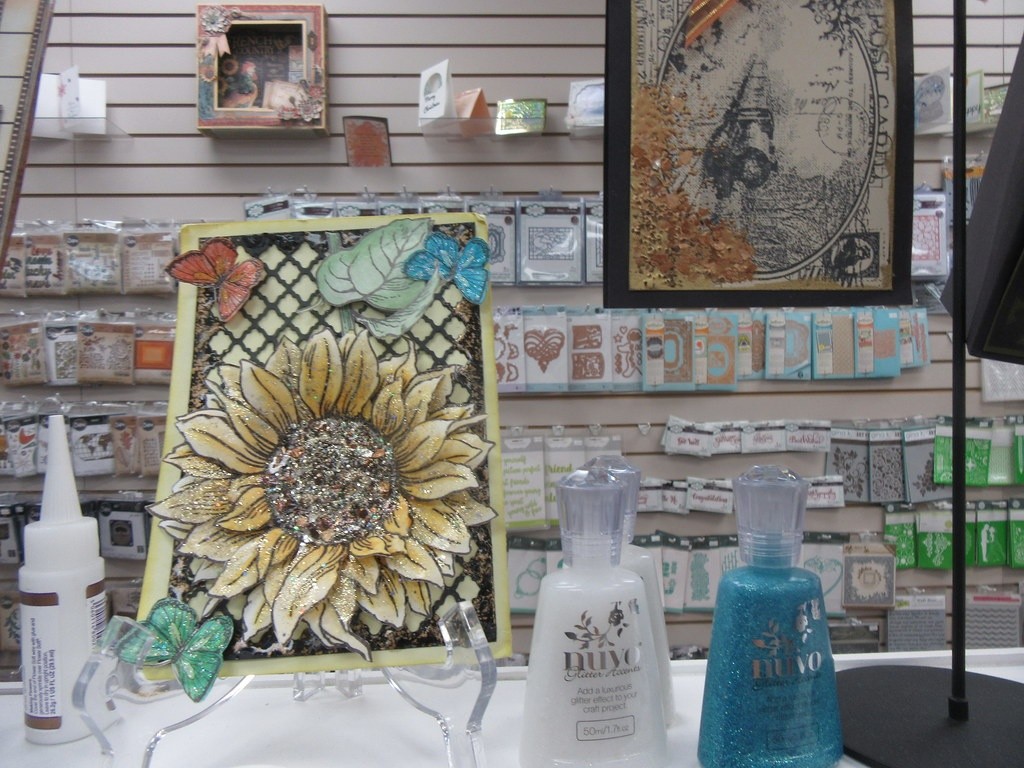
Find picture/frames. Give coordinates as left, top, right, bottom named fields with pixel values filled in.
left=603, top=0, right=916, bottom=307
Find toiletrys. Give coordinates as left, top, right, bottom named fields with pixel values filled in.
left=696, top=462, right=845, bottom=767
left=522, top=468, right=668, bottom=767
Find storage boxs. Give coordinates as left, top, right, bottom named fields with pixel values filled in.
left=196, top=3, right=331, bottom=140
